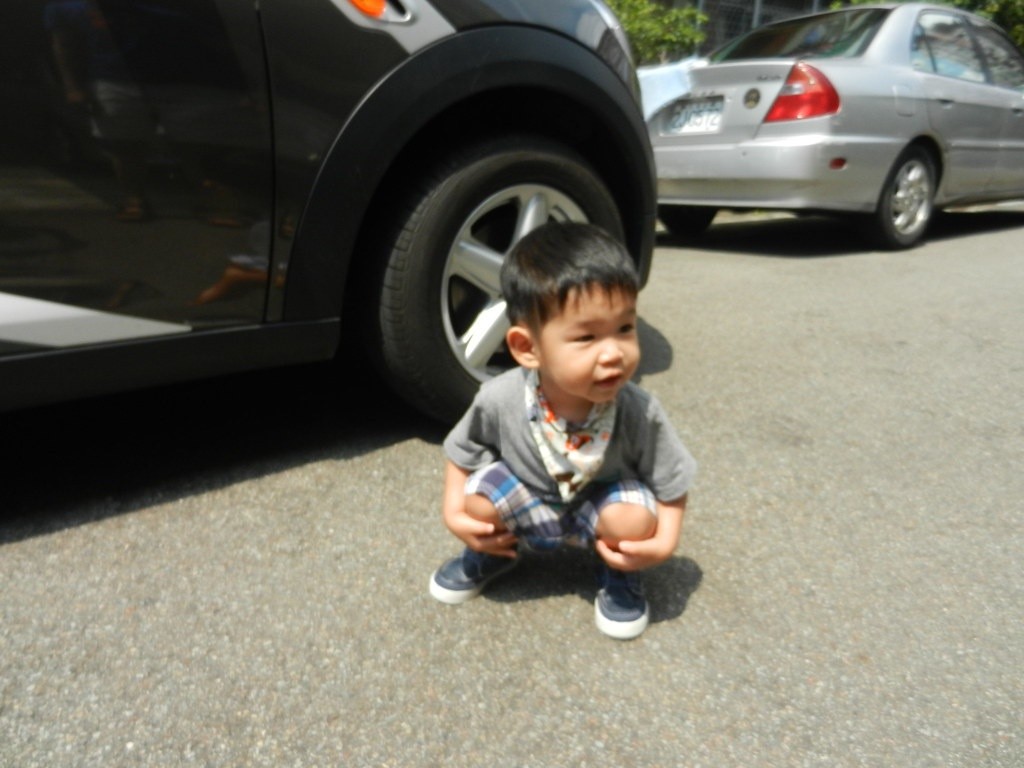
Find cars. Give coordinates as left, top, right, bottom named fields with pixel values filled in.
left=0, top=0, right=659, bottom=429
left=644, top=5, right=1024, bottom=250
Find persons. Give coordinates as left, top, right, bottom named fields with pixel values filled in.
left=429, top=221, right=689, bottom=640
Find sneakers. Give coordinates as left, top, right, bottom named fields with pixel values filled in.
left=595, top=568, right=651, bottom=639
left=429, top=543, right=521, bottom=604
